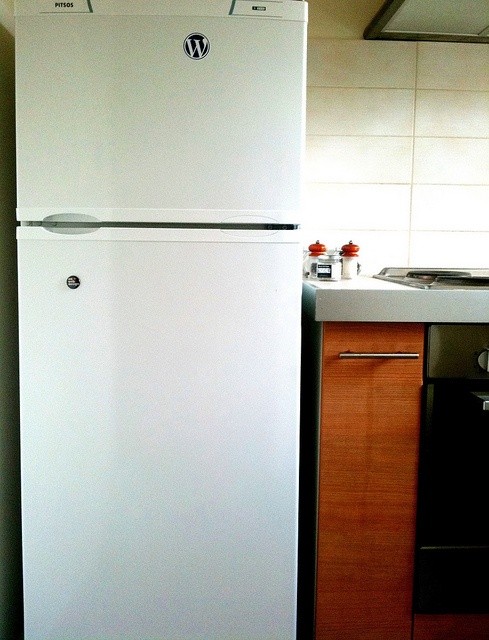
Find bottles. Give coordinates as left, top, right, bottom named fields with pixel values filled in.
left=316, top=255, right=341, bottom=282
left=308, top=240, right=327, bottom=280
left=341, top=240, right=359, bottom=280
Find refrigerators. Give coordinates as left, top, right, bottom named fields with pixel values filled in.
left=11, top=1, right=309, bottom=640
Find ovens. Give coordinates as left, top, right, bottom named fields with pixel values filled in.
left=413, top=324, right=487, bottom=616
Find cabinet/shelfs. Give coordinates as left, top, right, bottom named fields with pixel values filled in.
left=302, top=322, right=424, bottom=640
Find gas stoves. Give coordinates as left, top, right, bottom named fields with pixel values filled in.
left=406, top=270, right=489, bottom=286
